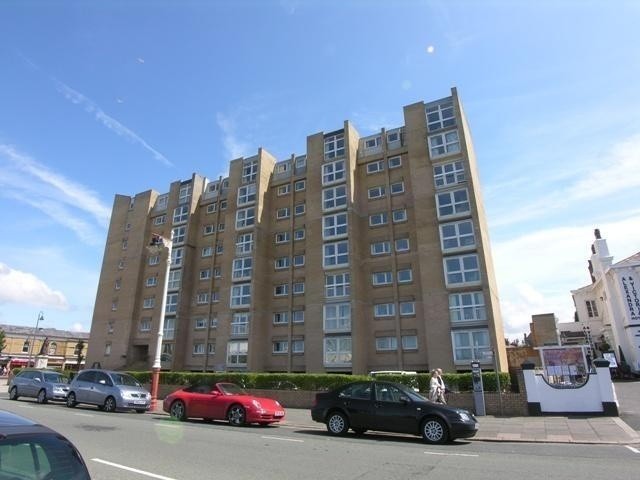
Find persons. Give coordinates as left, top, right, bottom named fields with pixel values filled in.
left=437, top=368, right=446, bottom=403
left=40, top=337, right=49, bottom=355
left=429, top=370, right=441, bottom=401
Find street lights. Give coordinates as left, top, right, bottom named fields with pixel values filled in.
left=145, top=229, right=175, bottom=411
left=27, top=311, right=45, bottom=368
left=483, top=346, right=505, bottom=418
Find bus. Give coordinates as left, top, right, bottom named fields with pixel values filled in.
left=4, top=357, right=35, bottom=375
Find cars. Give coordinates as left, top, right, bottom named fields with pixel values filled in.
left=0, top=407, right=90, bottom=479
left=66, top=368, right=152, bottom=414
left=310, top=381, right=479, bottom=444
left=8, top=367, right=70, bottom=403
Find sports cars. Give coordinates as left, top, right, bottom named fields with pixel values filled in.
left=163, top=382, right=286, bottom=427
